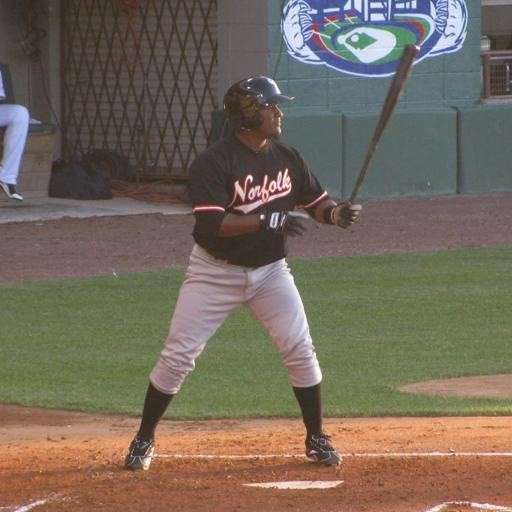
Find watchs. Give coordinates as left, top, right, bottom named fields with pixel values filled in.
left=258, top=212, right=266, bottom=232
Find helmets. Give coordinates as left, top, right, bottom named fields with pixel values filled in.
left=224, top=76, right=294, bottom=121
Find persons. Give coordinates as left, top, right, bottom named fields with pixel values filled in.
left=123, top=76, right=361, bottom=473
left=0, top=62, right=29, bottom=202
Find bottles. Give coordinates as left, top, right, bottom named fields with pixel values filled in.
left=480, top=35, right=490, bottom=51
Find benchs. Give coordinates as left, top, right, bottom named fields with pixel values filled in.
left=0, top=123, right=57, bottom=199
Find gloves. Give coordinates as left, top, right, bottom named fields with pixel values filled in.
left=333, top=200, right=361, bottom=228
left=260, top=210, right=309, bottom=237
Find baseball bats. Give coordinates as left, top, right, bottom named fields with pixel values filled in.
left=349, top=44, right=421, bottom=205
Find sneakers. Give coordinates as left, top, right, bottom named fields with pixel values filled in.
left=305, top=433, right=342, bottom=464
left=0, top=182, right=23, bottom=201
left=125, top=434, right=155, bottom=471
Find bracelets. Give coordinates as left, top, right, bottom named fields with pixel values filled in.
left=323, top=205, right=337, bottom=225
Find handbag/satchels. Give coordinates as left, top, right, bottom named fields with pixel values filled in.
left=48, top=156, right=113, bottom=200
